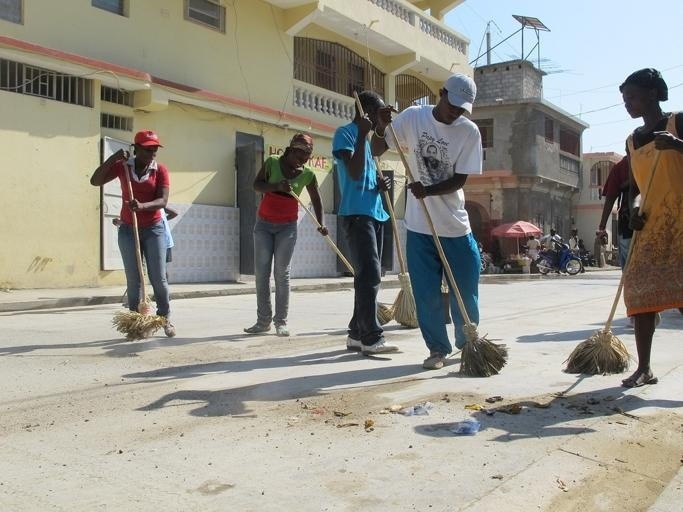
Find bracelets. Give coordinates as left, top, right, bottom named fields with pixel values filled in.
left=372, top=126, right=387, bottom=140
left=598, top=223, right=606, bottom=228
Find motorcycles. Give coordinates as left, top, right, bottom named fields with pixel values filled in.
left=475, top=241, right=489, bottom=274
left=535, top=238, right=583, bottom=276
left=579, top=249, right=597, bottom=267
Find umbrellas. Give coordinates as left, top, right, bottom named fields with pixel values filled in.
left=490, top=219, right=546, bottom=258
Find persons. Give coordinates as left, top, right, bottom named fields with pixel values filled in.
left=421, top=144, right=440, bottom=170
left=111, top=203, right=179, bottom=309
left=595, top=134, right=664, bottom=329
left=88, top=130, right=181, bottom=341
left=367, top=72, right=483, bottom=371
left=521, top=225, right=595, bottom=273
left=329, top=86, right=401, bottom=357
left=618, top=66, right=683, bottom=388
left=241, top=131, right=329, bottom=338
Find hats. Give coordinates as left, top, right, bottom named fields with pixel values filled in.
left=291, top=132, right=314, bottom=153
left=135, top=131, right=160, bottom=147
left=444, top=73, right=477, bottom=114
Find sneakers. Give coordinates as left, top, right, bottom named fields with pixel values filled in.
left=622, top=369, right=654, bottom=388
left=244, top=323, right=290, bottom=337
left=424, top=355, right=443, bottom=369
left=163, top=320, right=176, bottom=336
left=346, top=337, right=399, bottom=354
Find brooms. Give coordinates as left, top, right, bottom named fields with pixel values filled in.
left=352, top=89, right=421, bottom=330
left=387, top=122, right=510, bottom=378
left=559, top=149, right=664, bottom=376
left=289, top=189, right=395, bottom=327
left=110, top=160, right=176, bottom=343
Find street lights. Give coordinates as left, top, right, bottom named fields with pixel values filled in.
left=484, top=17, right=503, bottom=66
